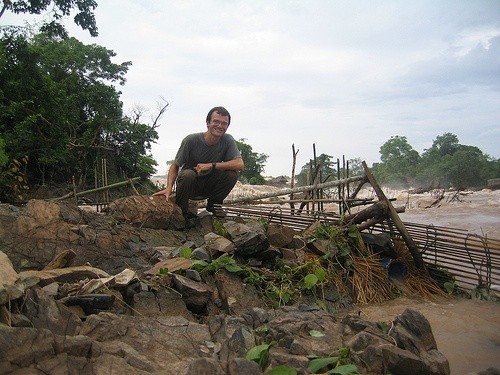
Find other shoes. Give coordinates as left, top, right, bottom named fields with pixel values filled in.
left=184, top=212, right=201, bottom=220
left=206, top=202, right=226, bottom=218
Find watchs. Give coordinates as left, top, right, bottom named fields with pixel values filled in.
left=212, top=161, right=217, bottom=171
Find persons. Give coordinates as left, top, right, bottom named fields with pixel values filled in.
left=150, top=105, right=245, bottom=227
